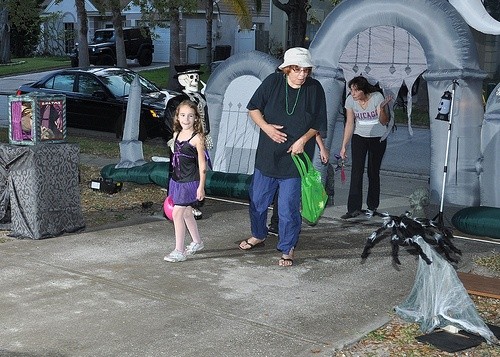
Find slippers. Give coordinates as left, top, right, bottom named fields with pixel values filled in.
left=239, top=236, right=266, bottom=250
left=280, top=257, right=293, bottom=266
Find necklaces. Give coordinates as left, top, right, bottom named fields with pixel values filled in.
left=286, top=75, right=300, bottom=115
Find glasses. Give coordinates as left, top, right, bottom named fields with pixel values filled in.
left=291, top=66, right=312, bottom=73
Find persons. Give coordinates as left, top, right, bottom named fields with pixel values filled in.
left=163, top=100, right=207, bottom=263
left=266, top=132, right=329, bottom=236
left=238, top=47, right=327, bottom=266
left=165, top=63, right=211, bottom=220
left=340, top=76, right=391, bottom=219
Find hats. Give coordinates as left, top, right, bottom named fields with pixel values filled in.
left=175, top=63, right=201, bottom=75
left=278, top=47, right=314, bottom=71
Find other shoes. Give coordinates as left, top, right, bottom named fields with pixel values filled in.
left=268, top=227, right=279, bottom=237
left=365, top=207, right=377, bottom=218
left=341, top=209, right=361, bottom=219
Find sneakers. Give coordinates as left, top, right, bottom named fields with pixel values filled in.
left=164, top=249, right=187, bottom=262
left=185, top=242, right=204, bottom=254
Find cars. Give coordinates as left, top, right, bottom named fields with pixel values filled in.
left=16, top=66, right=181, bottom=143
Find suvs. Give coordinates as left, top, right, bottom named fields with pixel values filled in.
left=71, top=26, right=155, bottom=66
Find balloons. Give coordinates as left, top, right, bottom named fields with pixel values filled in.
left=164, top=197, right=174, bottom=220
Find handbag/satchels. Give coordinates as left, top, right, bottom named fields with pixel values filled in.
left=291, top=151, right=328, bottom=226
